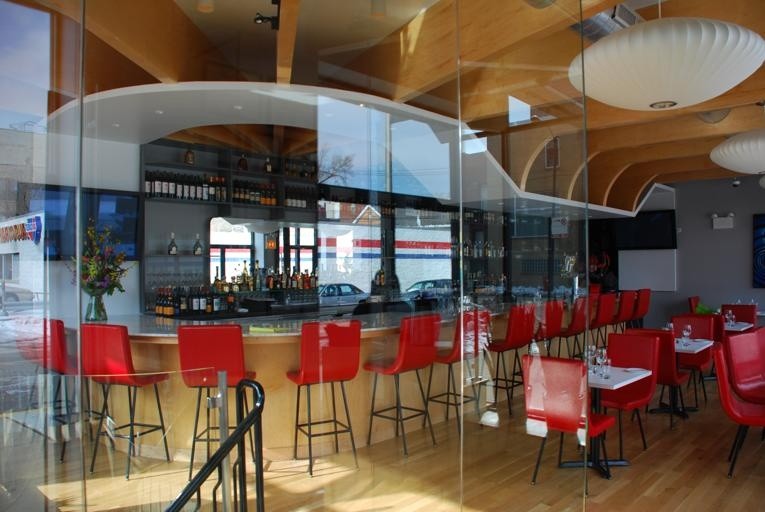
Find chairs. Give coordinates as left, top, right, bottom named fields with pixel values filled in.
left=285, top=319, right=362, bottom=477
left=360, top=311, right=441, bottom=454
left=177, top=323, right=256, bottom=481
left=12, top=315, right=94, bottom=465
left=79, top=320, right=171, bottom=482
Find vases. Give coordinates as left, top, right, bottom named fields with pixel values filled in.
left=80, top=287, right=107, bottom=323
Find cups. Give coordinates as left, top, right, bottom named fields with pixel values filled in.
left=600, top=358, right=612, bottom=376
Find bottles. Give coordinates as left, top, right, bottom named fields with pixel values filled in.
left=154, top=285, right=212, bottom=317
left=285, top=159, right=317, bottom=181
left=185, top=145, right=195, bottom=165
left=242, top=260, right=319, bottom=291
left=264, top=156, right=271, bottom=173
left=237, top=152, right=248, bottom=171
left=145, top=169, right=228, bottom=202
left=233, top=178, right=277, bottom=205
left=193, top=233, right=203, bottom=256
left=453, top=270, right=508, bottom=293
left=285, top=184, right=316, bottom=209
left=213, top=264, right=242, bottom=314
left=168, top=232, right=177, bottom=255
left=450, top=237, right=504, bottom=257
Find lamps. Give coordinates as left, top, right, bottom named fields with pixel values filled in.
left=567, top=0, right=764, bottom=111
left=710, top=127, right=765, bottom=175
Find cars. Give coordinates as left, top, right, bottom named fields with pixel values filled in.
left=317, top=283, right=371, bottom=316
left=407, top=280, right=453, bottom=300
left=0, top=286, right=33, bottom=312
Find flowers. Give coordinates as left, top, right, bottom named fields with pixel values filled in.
left=55, top=212, right=137, bottom=321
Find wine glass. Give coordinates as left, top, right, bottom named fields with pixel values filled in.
left=725, top=310, right=733, bottom=328
left=594, top=346, right=609, bottom=379
left=584, top=344, right=596, bottom=373
left=682, top=324, right=692, bottom=346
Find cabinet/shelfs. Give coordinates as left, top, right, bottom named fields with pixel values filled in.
left=456, top=209, right=509, bottom=294
left=321, top=197, right=454, bottom=300
left=509, top=213, right=553, bottom=295
left=138, top=140, right=319, bottom=313
